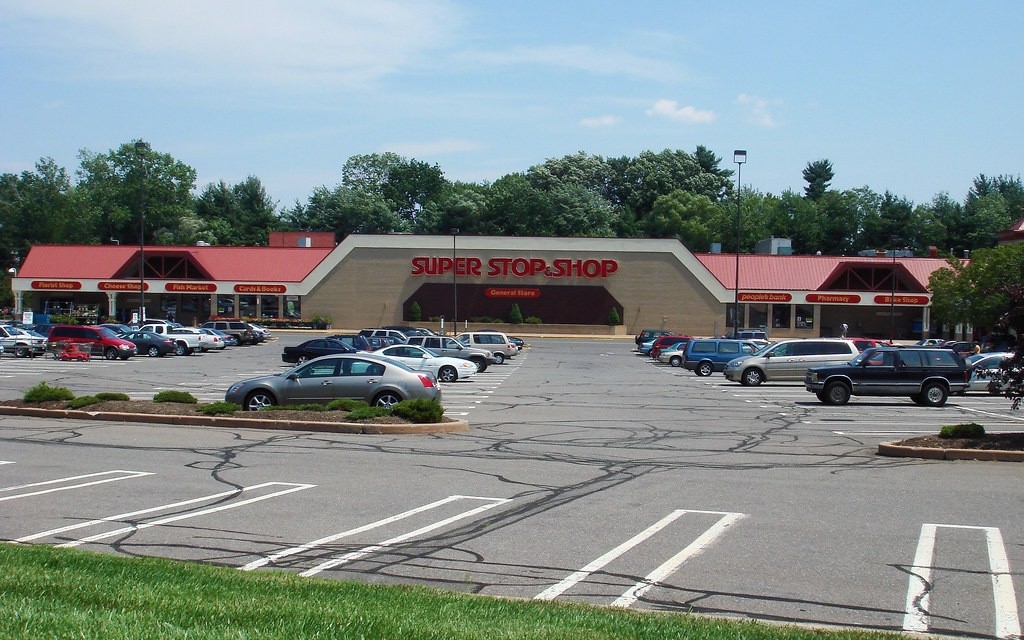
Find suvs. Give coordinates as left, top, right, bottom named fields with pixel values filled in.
left=803, top=345, right=973, bottom=405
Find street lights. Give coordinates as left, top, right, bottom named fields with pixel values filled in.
left=734, top=149, right=747, bottom=344
left=135, top=141, right=146, bottom=336
left=450, top=227, right=460, bottom=337
left=889, top=234, right=900, bottom=344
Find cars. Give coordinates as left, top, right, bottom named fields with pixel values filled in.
left=635, top=328, right=894, bottom=387
left=224, top=352, right=442, bottom=412
left=281, top=325, right=525, bottom=383
left=915, top=338, right=1024, bottom=392
left=0, top=317, right=272, bottom=358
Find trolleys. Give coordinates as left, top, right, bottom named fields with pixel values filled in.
left=45, top=338, right=95, bottom=362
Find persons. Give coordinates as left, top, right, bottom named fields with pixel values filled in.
left=840, top=321, right=848, bottom=338
left=167, top=312, right=174, bottom=323
left=970, top=341, right=980, bottom=355
